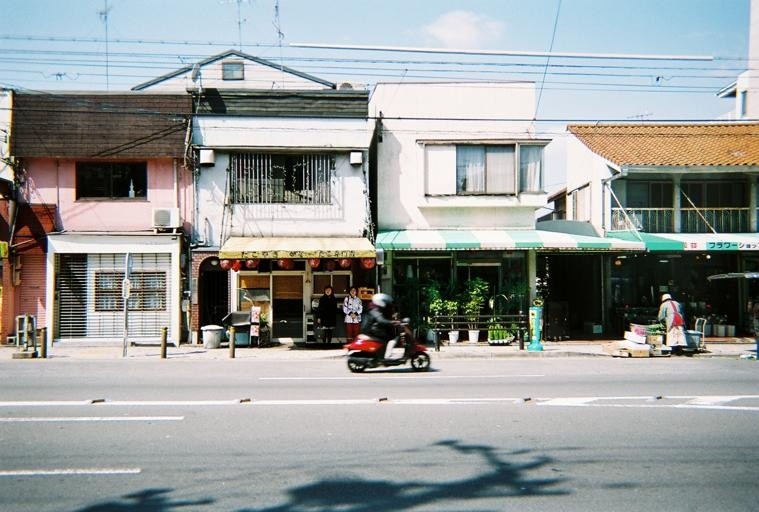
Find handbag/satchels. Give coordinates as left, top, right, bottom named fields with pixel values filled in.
left=315, top=327, right=325, bottom=338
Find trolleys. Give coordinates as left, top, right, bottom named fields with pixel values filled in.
left=680, top=317, right=708, bottom=355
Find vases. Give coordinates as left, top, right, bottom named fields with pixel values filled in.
left=697, top=322, right=736, bottom=337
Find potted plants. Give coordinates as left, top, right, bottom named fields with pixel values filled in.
left=260, top=313, right=268, bottom=328
left=392, top=271, right=531, bottom=344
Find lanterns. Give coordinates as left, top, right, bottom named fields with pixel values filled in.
left=220, top=258, right=375, bottom=272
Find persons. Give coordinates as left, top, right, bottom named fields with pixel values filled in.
left=317, top=286, right=337, bottom=344
left=752, top=302, right=759, bottom=360
left=361, top=293, right=401, bottom=360
left=657, top=293, right=689, bottom=355
left=342, top=286, right=363, bottom=341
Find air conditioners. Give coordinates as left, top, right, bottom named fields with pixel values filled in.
left=152, top=207, right=181, bottom=228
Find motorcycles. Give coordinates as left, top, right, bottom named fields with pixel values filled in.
left=343, top=311, right=433, bottom=375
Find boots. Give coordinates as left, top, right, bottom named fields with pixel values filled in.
left=385, top=341, right=397, bottom=359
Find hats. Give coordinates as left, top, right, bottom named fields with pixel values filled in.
left=661, top=293, right=671, bottom=301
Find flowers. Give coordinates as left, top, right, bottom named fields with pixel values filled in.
left=622, top=291, right=727, bottom=324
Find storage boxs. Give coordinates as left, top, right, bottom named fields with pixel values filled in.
left=646, top=335, right=664, bottom=345
left=684, top=330, right=701, bottom=347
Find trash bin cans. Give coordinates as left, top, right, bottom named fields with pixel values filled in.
left=234, top=328, right=249, bottom=346
left=200, top=325, right=224, bottom=349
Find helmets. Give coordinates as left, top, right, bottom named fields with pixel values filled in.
left=372, top=293, right=394, bottom=308
